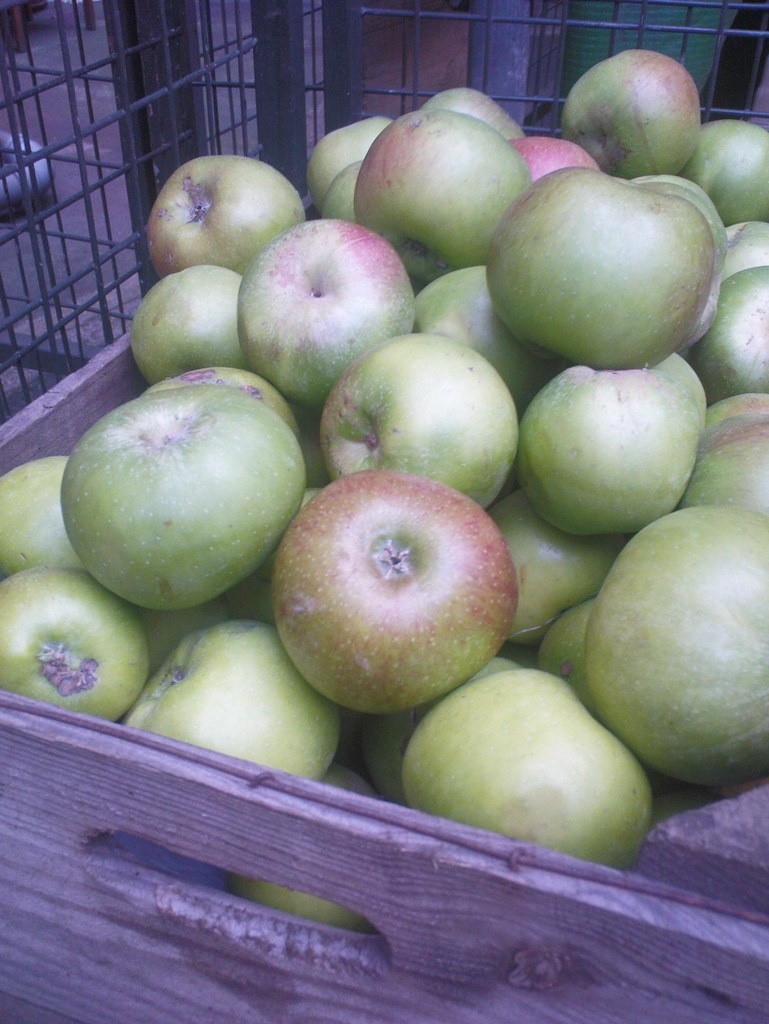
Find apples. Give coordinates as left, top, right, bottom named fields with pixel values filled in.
left=0, top=48, right=769, bottom=931
